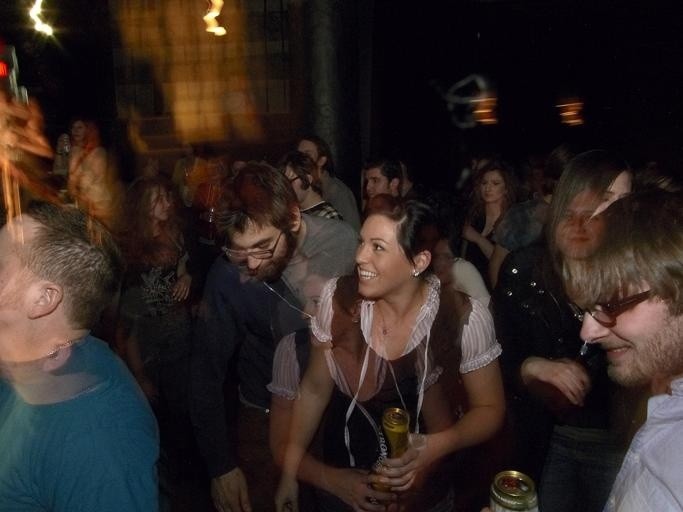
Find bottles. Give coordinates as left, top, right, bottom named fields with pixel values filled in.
left=204, top=206, right=218, bottom=241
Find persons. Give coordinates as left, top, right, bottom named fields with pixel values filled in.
left=1, top=85, right=683, bottom=512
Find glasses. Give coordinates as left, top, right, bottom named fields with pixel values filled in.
left=567, top=289, right=657, bottom=327
left=221, top=231, right=283, bottom=260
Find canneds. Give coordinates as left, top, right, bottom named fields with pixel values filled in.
left=379, top=407, right=410, bottom=458
left=488, top=471, right=543, bottom=512
